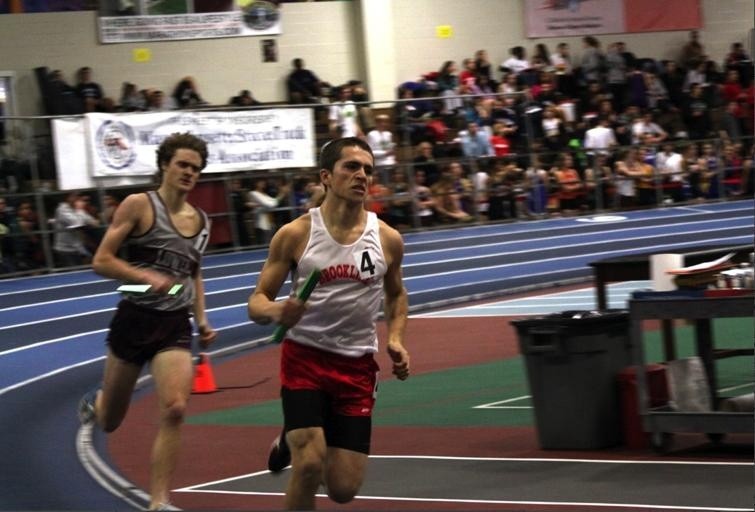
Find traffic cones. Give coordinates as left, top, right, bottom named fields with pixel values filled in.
left=190, top=350, right=220, bottom=395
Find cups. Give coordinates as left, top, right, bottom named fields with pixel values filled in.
left=648, top=251, right=683, bottom=291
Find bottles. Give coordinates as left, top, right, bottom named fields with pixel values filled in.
left=713, top=262, right=755, bottom=288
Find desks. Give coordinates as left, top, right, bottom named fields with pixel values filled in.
left=587, top=243, right=755, bottom=442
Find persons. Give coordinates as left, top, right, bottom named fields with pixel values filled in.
left=77, top=132, right=218, bottom=511
left=248, top=139, right=411, bottom=511
left=0, top=28, right=755, bottom=269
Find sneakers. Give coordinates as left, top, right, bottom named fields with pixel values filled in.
left=267, top=428, right=291, bottom=471
left=77, top=384, right=103, bottom=424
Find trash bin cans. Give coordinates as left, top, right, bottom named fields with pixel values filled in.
left=508, top=309, right=631, bottom=451
